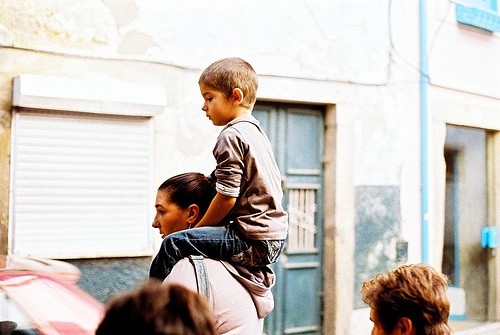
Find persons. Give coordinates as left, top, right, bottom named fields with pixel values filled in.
left=150, top=170, right=277, bottom=334
left=146, top=56, right=291, bottom=287
left=359, top=263, right=453, bottom=335
left=94, top=281, right=221, bottom=335
